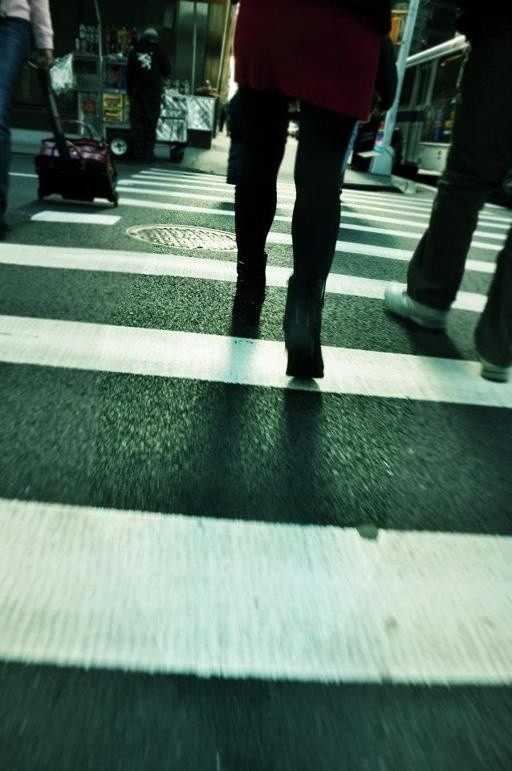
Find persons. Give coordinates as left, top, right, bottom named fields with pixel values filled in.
left=377, top=0, right=512, bottom=384
left=192, top=80, right=221, bottom=99
left=104, top=37, right=139, bottom=91
left=220, top=3, right=401, bottom=380
left=0, top=0, right=60, bottom=243
left=123, top=26, right=171, bottom=170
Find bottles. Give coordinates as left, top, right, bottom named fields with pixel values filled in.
left=77, top=23, right=143, bottom=60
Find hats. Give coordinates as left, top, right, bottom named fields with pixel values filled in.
left=144, top=27, right=159, bottom=41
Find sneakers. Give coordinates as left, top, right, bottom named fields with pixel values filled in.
left=383, top=280, right=451, bottom=330
left=480, top=356, right=511, bottom=384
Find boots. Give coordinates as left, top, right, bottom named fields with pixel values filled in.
left=280, top=270, right=327, bottom=379
left=233, top=249, right=267, bottom=307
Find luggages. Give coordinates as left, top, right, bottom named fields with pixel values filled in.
left=25, top=56, right=120, bottom=207
